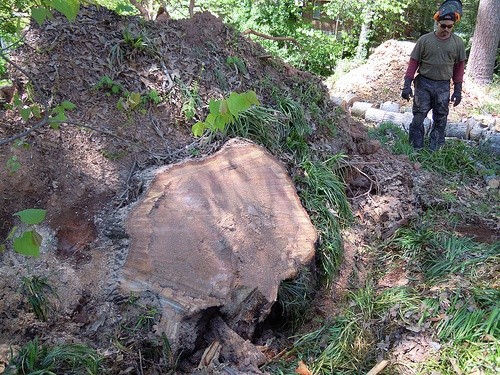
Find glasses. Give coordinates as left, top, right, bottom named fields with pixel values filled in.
left=438, top=21, right=455, bottom=29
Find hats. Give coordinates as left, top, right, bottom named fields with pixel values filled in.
left=433, top=0, right=463, bottom=22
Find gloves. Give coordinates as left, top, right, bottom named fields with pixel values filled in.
left=451, top=82, right=462, bottom=107
left=401, top=78, right=414, bottom=102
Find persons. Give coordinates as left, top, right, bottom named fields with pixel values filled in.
left=401, top=0, right=467, bottom=151
